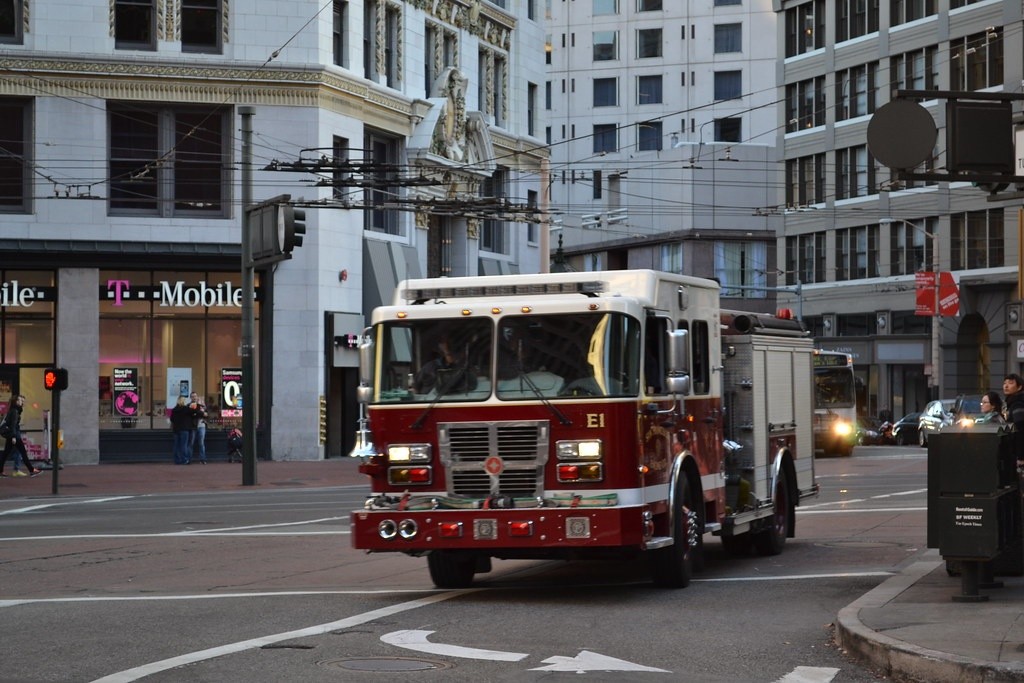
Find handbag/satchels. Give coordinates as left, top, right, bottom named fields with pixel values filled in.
left=0, top=423, right=10, bottom=438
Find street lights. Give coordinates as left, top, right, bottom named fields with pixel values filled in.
left=877, top=216, right=943, bottom=399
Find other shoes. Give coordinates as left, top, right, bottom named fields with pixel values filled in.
left=199, top=460, right=206, bottom=463
left=185, top=461, right=191, bottom=464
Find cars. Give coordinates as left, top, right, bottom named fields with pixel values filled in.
left=892, top=411, right=923, bottom=445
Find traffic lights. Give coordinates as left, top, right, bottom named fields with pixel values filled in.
left=43, top=368, right=69, bottom=391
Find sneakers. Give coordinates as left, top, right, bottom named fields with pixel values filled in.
left=30, top=470, right=43, bottom=477
left=12, top=472, right=27, bottom=477
left=1, top=473, right=9, bottom=478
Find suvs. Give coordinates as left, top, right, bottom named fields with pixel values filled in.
left=918, top=399, right=955, bottom=446
left=949, top=393, right=986, bottom=427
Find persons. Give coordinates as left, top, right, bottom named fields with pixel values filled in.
left=410, top=330, right=477, bottom=396
left=979, top=391, right=1007, bottom=425
left=0, top=395, right=44, bottom=478
left=7, top=395, right=27, bottom=477
left=878, top=403, right=894, bottom=425
left=170, top=396, right=208, bottom=465
left=185, top=391, right=208, bottom=465
left=1002, top=373, right=1024, bottom=433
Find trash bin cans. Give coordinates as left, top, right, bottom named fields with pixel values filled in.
left=926, top=422, right=1016, bottom=602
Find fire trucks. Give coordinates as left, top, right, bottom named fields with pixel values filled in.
left=347, top=266, right=821, bottom=591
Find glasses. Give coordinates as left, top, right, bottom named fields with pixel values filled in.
left=981, top=401, right=990, bottom=403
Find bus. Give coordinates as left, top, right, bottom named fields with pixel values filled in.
left=811, top=349, right=863, bottom=458
